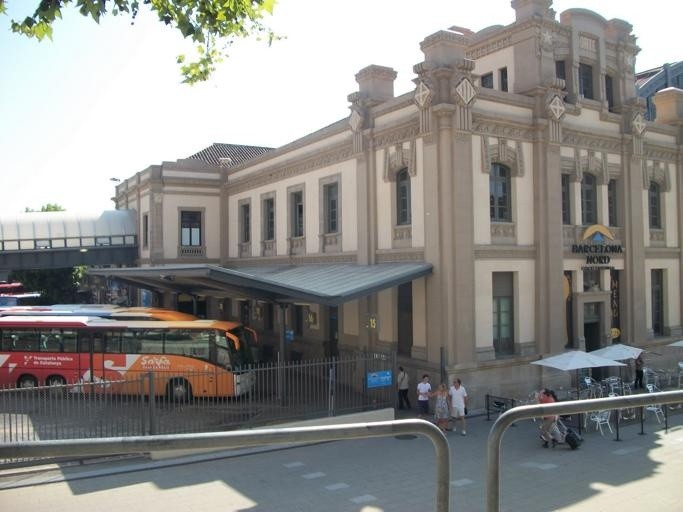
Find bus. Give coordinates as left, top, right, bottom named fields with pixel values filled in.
left=0, top=314, right=261, bottom=406
left=0, top=291, right=46, bottom=307
left=0, top=280, right=25, bottom=294
left=0, top=302, right=197, bottom=322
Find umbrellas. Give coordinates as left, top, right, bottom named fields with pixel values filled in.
left=665, top=340, right=683, bottom=347
left=528, top=350, right=628, bottom=434
left=587, top=344, right=645, bottom=394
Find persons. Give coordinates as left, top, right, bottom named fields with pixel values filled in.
left=396, top=366, right=411, bottom=410
left=415, top=375, right=432, bottom=420
left=535, top=388, right=557, bottom=448
left=449, top=378, right=468, bottom=436
left=547, top=390, right=561, bottom=444
left=635, top=355, right=644, bottom=389
left=432, top=383, right=450, bottom=433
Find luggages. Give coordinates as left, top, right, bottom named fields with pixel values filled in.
left=558, top=419, right=582, bottom=449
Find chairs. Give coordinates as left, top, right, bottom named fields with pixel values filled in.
left=504, top=360, right=682, bottom=437
